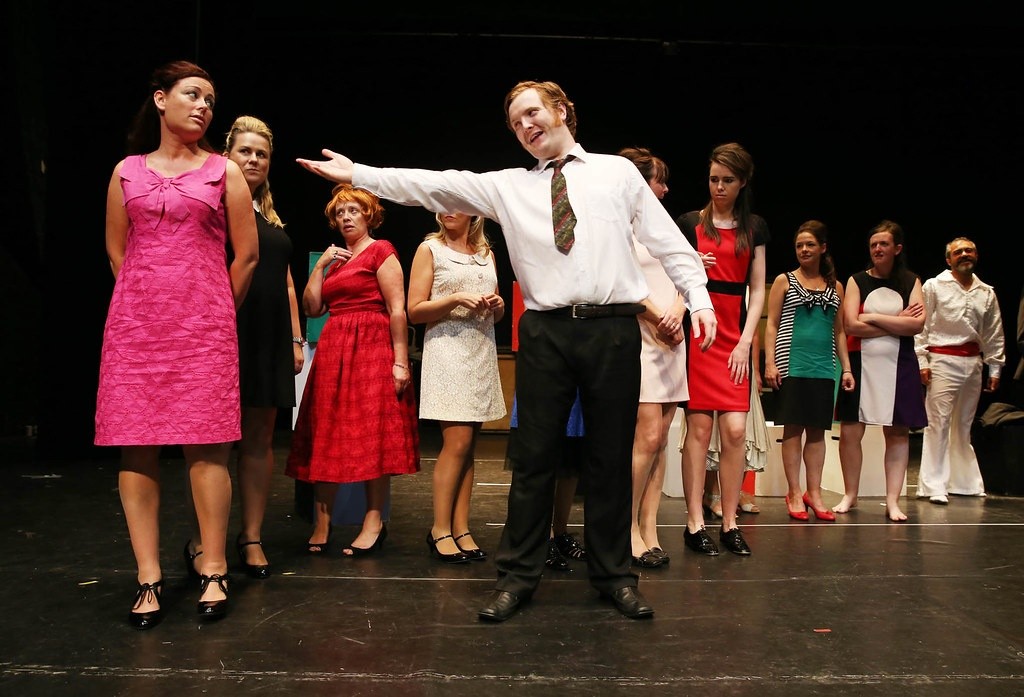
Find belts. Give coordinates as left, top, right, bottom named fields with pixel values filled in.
left=555, top=302, right=647, bottom=319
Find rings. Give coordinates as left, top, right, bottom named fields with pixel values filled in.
left=335, top=251, right=338, bottom=254
left=334, top=255, right=336, bottom=258
left=672, top=325, right=675, bottom=328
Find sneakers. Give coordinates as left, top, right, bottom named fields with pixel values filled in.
left=555, top=533, right=588, bottom=560
left=545, top=538, right=569, bottom=568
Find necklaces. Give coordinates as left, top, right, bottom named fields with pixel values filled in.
left=798, top=268, right=823, bottom=292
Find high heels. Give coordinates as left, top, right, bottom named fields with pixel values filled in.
left=802, top=491, right=836, bottom=521
left=306, top=523, right=332, bottom=553
left=197, top=570, right=230, bottom=615
left=236, top=532, right=271, bottom=578
left=453, top=532, right=486, bottom=559
left=738, top=491, right=760, bottom=513
left=702, top=492, right=739, bottom=518
left=342, top=522, right=387, bottom=558
left=786, top=495, right=810, bottom=520
left=184, top=540, right=206, bottom=578
left=426, top=532, right=471, bottom=562
left=128, top=574, right=164, bottom=629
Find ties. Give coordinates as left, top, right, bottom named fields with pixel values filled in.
left=546, top=155, right=577, bottom=255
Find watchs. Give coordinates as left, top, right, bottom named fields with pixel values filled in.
left=292, top=337, right=304, bottom=344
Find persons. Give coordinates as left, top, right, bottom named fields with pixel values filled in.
left=283, top=182, right=422, bottom=554
left=186, top=114, right=303, bottom=579
left=296, top=80, right=718, bottom=623
left=93, top=62, right=260, bottom=631
left=406, top=205, right=508, bottom=564
left=678, top=285, right=773, bottom=520
left=509, top=379, right=585, bottom=571
left=831, top=219, right=927, bottom=521
left=613, top=146, right=692, bottom=568
left=915, top=234, right=1006, bottom=504
left=676, top=143, right=766, bottom=553
left=765, top=219, right=855, bottom=522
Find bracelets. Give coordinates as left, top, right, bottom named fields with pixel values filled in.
left=843, top=371, right=852, bottom=373
left=394, top=363, right=410, bottom=370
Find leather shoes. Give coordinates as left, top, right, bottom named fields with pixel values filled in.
left=599, top=586, right=655, bottom=617
left=649, top=547, right=670, bottom=561
left=477, top=590, right=523, bottom=620
left=632, top=551, right=662, bottom=567
left=684, top=526, right=720, bottom=555
left=720, top=527, right=751, bottom=554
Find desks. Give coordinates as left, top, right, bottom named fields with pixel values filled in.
left=482, top=353, right=518, bottom=435
